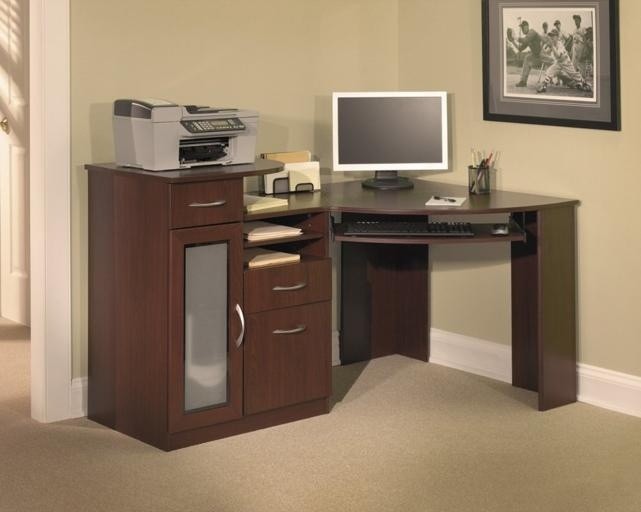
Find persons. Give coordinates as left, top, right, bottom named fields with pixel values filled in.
left=505, top=11, right=594, bottom=98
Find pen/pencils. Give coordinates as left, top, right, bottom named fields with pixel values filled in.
left=470, top=151, right=493, bottom=195
left=434, top=196, right=456, bottom=202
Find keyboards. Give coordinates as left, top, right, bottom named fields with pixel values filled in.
left=346, top=221, right=475, bottom=236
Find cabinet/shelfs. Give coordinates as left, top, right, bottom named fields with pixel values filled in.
left=82, top=156, right=331, bottom=452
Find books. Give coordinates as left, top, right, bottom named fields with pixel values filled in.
left=240, top=219, right=304, bottom=242
left=243, top=247, right=303, bottom=270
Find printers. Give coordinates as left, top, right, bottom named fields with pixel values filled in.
left=111, top=96, right=261, bottom=172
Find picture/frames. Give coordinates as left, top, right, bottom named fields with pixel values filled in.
left=480, top=0, right=619, bottom=133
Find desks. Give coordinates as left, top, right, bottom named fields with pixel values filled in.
left=333, top=175, right=580, bottom=413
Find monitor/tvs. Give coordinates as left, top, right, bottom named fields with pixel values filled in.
left=332, top=90, right=450, bottom=190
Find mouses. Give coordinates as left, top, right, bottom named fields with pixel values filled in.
left=489, top=221, right=509, bottom=236
